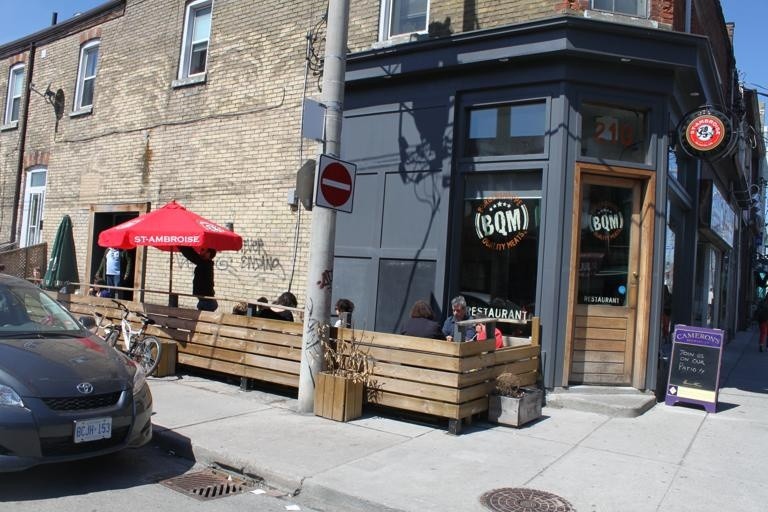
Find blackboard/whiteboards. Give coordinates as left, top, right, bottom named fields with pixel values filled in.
left=665, top=324, right=724, bottom=415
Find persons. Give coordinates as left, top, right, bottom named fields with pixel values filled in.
left=59, top=246, right=132, bottom=298
left=255, top=297, right=268, bottom=317
left=708, top=287, right=714, bottom=320
left=472, top=312, right=504, bottom=349
left=233, top=300, right=247, bottom=315
left=33, top=266, right=42, bottom=288
left=177, top=245, right=218, bottom=311
left=400, top=300, right=442, bottom=339
left=756, top=293, right=768, bottom=352
left=260, top=292, right=297, bottom=322
left=334, top=298, right=354, bottom=327
left=442, top=296, right=476, bottom=343
left=0, top=264, right=6, bottom=274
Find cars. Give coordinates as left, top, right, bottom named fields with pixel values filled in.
left=580, top=269, right=626, bottom=305
left=459, top=290, right=533, bottom=342
left=0, top=272, right=156, bottom=474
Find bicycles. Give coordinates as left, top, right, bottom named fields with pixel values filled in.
left=88, top=309, right=113, bottom=340
left=107, top=298, right=163, bottom=377
left=23, top=299, right=72, bottom=330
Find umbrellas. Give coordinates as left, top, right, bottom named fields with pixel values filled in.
left=97, top=198, right=250, bottom=329
left=40, top=215, right=80, bottom=290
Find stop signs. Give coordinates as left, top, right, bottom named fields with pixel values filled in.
left=314, top=153, right=357, bottom=214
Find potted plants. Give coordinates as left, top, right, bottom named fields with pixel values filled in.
left=304, top=319, right=374, bottom=422
left=487, top=371, right=544, bottom=428
